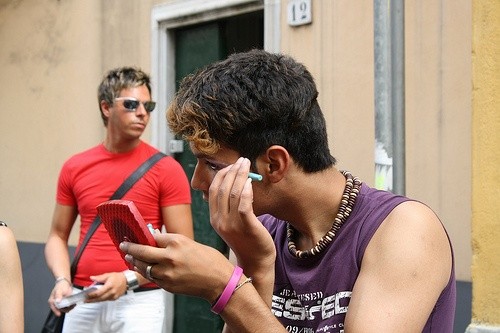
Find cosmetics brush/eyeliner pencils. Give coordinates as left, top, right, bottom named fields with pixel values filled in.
left=217, top=162, right=262, bottom=181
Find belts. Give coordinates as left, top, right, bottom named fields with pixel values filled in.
left=73, top=285, right=161, bottom=294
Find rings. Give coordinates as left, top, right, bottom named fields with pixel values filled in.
left=146, top=263, right=152, bottom=281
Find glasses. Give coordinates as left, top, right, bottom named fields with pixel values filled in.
left=115, top=97, right=156, bottom=112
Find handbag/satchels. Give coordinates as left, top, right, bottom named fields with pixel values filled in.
left=41, top=307, right=68, bottom=333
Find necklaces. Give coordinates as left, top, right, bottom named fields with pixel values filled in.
left=287, top=170, right=361, bottom=261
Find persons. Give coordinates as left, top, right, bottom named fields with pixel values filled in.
left=0, top=221, right=25, bottom=333
left=120, top=49, right=457, bottom=333
left=43, top=66, right=194, bottom=333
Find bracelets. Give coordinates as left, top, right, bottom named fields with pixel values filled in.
left=124, top=269, right=140, bottom=290
left=55, top=277, right=72, bottom=287
left=211, top=266, right=251, bottom=314
